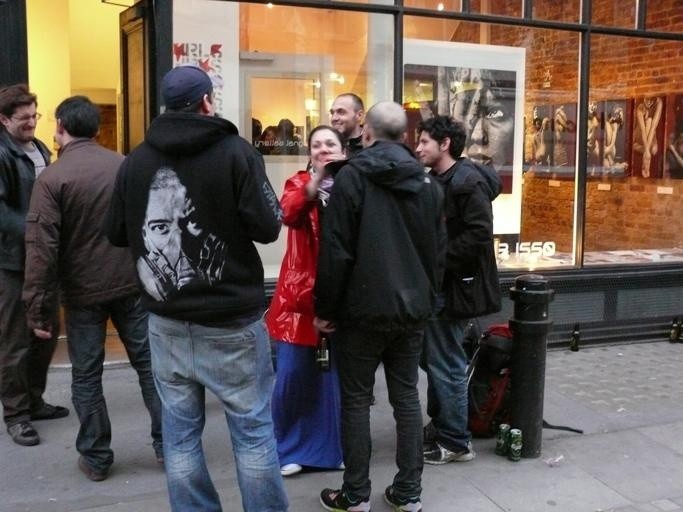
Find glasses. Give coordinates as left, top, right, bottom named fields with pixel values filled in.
left=13, top=112, right=43, bottom=122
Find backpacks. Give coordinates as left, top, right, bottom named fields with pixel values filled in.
left=465, top=323, right=514, bottom=437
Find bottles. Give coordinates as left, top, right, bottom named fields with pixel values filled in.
left=669, top=318, right=678, bottom=342
left=315, top=329, right=331, bottom=371
left=569, top=323, right=581, bottom=351
left=678, top=320, right=683, bottom=342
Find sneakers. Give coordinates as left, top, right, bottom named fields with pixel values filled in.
left=8, top=422, right=40, bottom=446
left=422, top=423, right=438, bottom=443
left=424, top=441, right=476, bottom=464
left=31, top=404, right=69, bottom=421
left=384, top=486, right=423, bottom=512
left=320, top=488, right=371, bottom=511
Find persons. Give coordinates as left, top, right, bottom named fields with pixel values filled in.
left=269, top=92, right=365, bottom=473
left=107, top=66, right=291, bottom=510
left=415, top=117, right=501, bottom=466
left=252, top=117, right=298, bottom=154
left=447, top=68, right=513, bottom=181
left=313, top=101, right=439, bottom=512
left=532, top=98, right=664, bottom=178
left=22, top=96, right=164, bottom=480
left=0, top=82, right=70, bottom=447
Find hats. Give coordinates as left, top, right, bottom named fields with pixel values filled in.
left=161, top=66, right=212, bottom=110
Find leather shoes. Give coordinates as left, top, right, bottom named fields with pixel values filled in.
left=78, top=456, right=106, bottom=480
left=280, top=463, right=302, bottom=476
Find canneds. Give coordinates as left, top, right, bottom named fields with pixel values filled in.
left=509, top=429, right=523, bottom=462
left=496, top=424, right=511, bottom=456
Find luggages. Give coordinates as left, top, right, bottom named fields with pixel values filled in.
left=422, top=319, right=482, bottom=415
